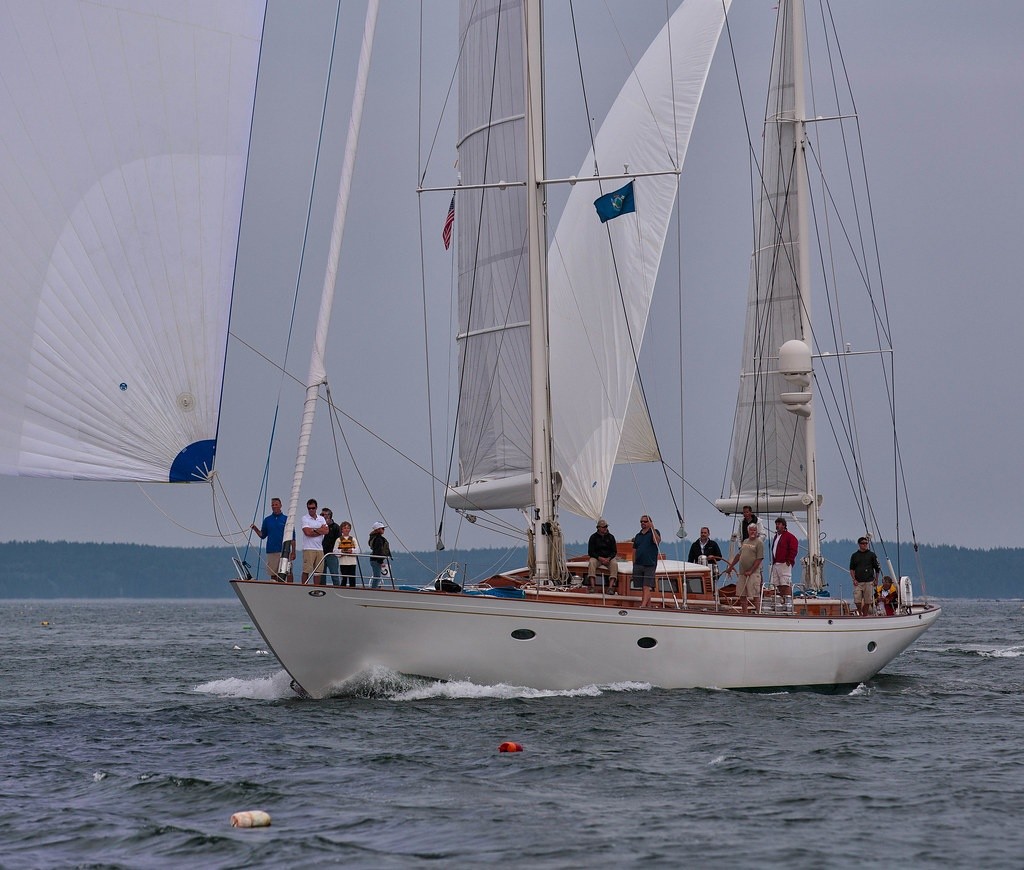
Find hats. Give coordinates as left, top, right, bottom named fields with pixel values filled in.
left=596, top=520, right=609, bottom=528
left=858, top=537, right=867, bottom=543
left=371, top=522, right=386, bottom=530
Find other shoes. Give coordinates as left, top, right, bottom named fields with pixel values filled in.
left=607, top=588, right=614, bottom=594
left=589, top=588, right=594, bottom=593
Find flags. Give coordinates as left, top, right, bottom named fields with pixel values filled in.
left=594, top=180, right=637, bottom=223
left=442, top=196, right=455, bottom=250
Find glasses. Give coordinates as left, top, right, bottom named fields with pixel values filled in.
left=320, top=513, right=329, bottom=517
left=859, top=542, right=867, bottom=544
left=380, top=528, right=384, bottom=530
left=640, top=521, right=649, bottom=523
left=602, top=526, right=607, bottom=528
left=308, top=507, right=315, bottom=510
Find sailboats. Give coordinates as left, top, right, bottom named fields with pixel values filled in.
left=0, top=3, right=943, bottom=697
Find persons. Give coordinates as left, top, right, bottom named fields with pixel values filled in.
left=870, top=576, right=898, bottom=616
left=769, top=517, right=799, bottom=604
left=368, top=522, right=389, bottom=588
left=726, top=523, right=765, bottom=614
left=688, top=527, right=722, bottom=581
left=632, top=515, right=661, bottom=608
left=850, top=537, right=880, bottom=616
left=588, top=520, right=618, bottom=595
left=737, top=505, right=766, bottom=582
left=320, top=508, right=340, bottom=586
left=301, top=499, right=329, bottom=584
left=333, top=522, right=360, bottom=587
left=251, top=497, right=297, bottom=582
